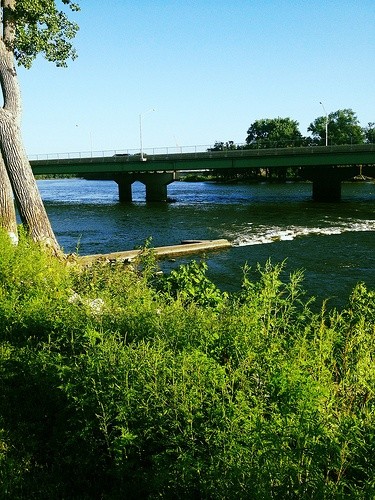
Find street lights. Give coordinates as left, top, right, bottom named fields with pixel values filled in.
left=140, top=109, right=156, bottom=160
left=319, top=101, right=328, bottom=146
